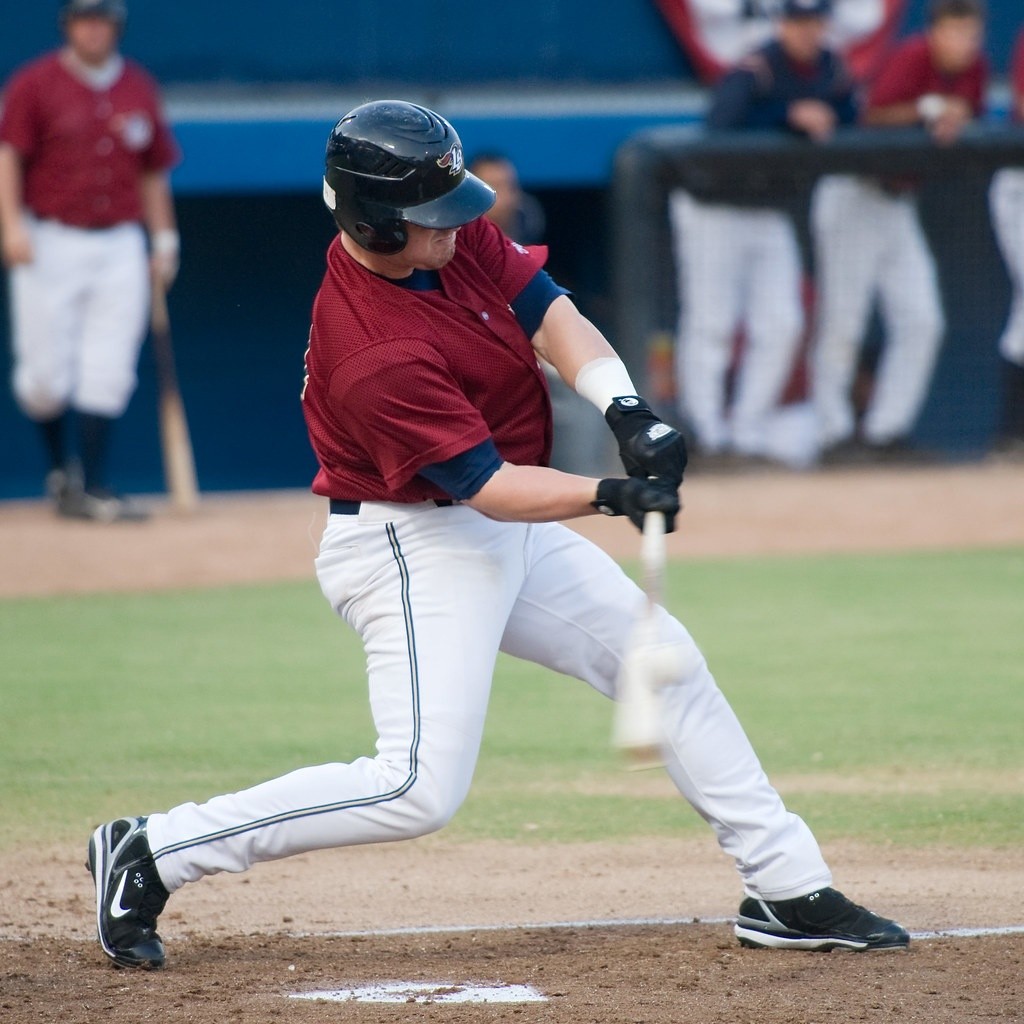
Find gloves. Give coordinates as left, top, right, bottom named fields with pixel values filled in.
left=604, top=393, right=688, bottom=489
left=591, top=475, right=681, bottom=533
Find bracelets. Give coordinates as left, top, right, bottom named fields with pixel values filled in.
left=151, top=231, right=181, bottom=253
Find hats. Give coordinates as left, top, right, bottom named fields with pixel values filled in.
left=779, top=1, right=831, bottom=19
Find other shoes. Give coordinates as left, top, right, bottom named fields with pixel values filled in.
left=46, top=454, right=138, bottom=519
left=816, top=434, right=927, bottom=466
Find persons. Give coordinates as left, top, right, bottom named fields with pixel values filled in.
left=809, top=0, right=1024, bottom=468
left=89, top=98, right=911, bottom=969
left=668, top=0, right=863, bottom=464
left=470, top=150, right=544, bottom=252
left=0, top=3, right=181, bottom=521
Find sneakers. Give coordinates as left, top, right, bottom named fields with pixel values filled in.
left=733, top=887, right=910, bottom=953
left=83, top=815, right=170, bottom=971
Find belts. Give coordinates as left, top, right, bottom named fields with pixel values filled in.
left=329, top=497, right=453, bottom=515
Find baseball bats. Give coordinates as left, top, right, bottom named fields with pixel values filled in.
left=612, top=509, right=674, bottom=766
left=147, top=266, right=201, bottom=522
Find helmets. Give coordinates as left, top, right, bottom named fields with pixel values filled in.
left=58, top=0, right=131, bottom=30
left=323, top=98, right=497, bottom=257
